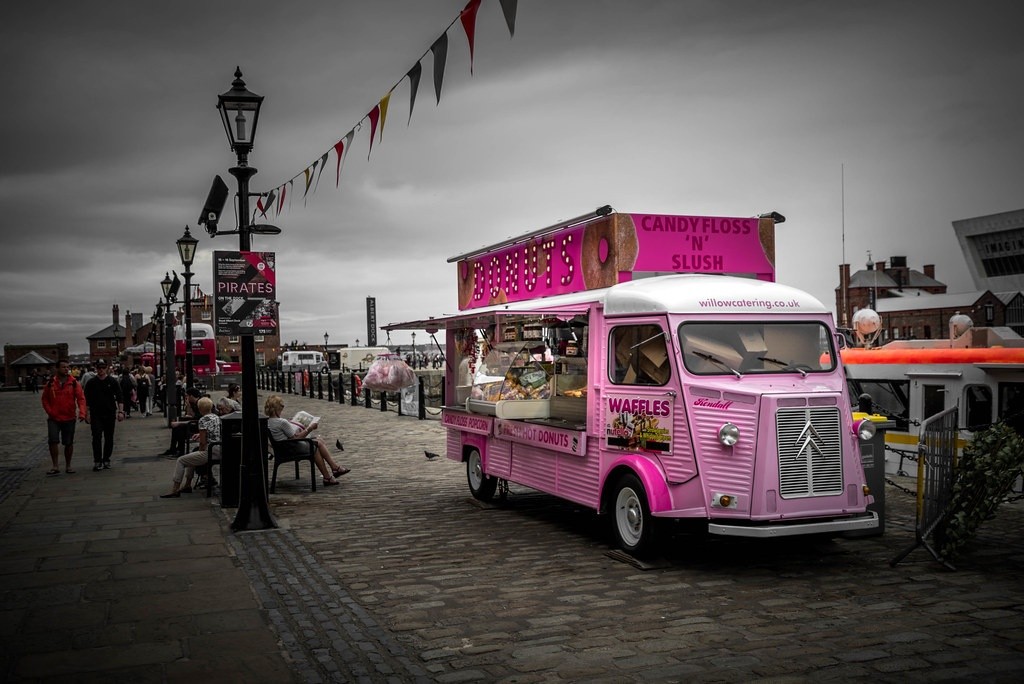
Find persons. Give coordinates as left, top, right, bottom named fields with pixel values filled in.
left=16, top=374, right=24, bottom=391
left=405, top=351, right=444, bottom=370
left=80, top=367, right=95, bottom=389
left=42, top=358, right=86, bottom=476
left=265, top=395, right=351, bottom=485
left=29, top=369, right=45, bottom=393
left=72, top=366, right=80, bottom=376
left=396, top=346, right=401, bottom=353
left=84, top=358, right=124, bottom=471
left=175, top=366, right=186, bottom=389
left=160, top=397, right=222, bottom=498
left=217, top=383, right=241, bottom=414
left=110, top=364, right=155, bottom=418
left=158, top=388, right=203, bottom=459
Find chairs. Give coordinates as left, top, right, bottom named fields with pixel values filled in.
left=178, top=408, right=315, bottom=498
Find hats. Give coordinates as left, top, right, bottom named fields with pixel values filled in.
left=186, top=387, right=200, bottom=398
left=95, top=358, right=107, bottom=365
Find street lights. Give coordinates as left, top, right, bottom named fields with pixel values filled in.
left=176, top=224, right=200, bottom=387
left=150, top=311, right=159, bottom=406
left=215, top=66, right=280, bottom=534
left=113, top=326, right=120, bottom=356
left=155, top=298, right=167, bottom=417
left=430, top=335, right=433, bottom=351
left=324, top=332, right=329, bottom=351
left=355, top=338, right=360, bottom=347
left=411, top=332, right=416, bottom=351
left=160, top=272, right=173, bottom=428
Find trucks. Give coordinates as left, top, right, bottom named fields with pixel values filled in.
left=380, top=204, right=879, bottom=557
left=282, top=351, right=329, bottom=374
left=339, top=347, right=392, bottom=374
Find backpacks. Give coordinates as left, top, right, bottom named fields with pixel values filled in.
left=137, top=374, right=147, bottom=392
left=120, top=377, right=130, bottom=392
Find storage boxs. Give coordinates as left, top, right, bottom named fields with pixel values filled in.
left=615, top=324, right=820, bottom=384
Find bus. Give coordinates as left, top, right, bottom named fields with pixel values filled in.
left=141, top=322, right=241, bottom=389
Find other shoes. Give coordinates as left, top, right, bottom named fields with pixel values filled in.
left=165, top=450, right=176, bottom=457
left=65, top=467, right=76, bottom=474
left=198, top=477, right=218, bottom=490
left=46, top=469, right=61, bottom=476
left=179, top=486, right=192, bottom=493
left=171, top=455, right=178, bottom=460
left=159, top=489, right=180, bottom=499
left=93, top=462, right=104, bottom=472
left=103, top=462, right=111, bottom=469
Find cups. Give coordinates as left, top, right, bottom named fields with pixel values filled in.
left=582, top=326, right=588, bottom=357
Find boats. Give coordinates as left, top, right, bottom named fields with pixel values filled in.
left=819, top=309, right=1024, bottom=492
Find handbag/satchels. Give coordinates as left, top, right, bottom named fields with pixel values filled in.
left=357, top=356, right=420, bottom=393
left=130, top=388, right=139, bottom=403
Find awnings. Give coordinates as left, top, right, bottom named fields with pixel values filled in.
left=379, top=305, right=589, bottom=371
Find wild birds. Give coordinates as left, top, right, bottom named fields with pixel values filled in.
left=336, top=439, right=344, bottom=451
left=423, top=451, right=439, bottom=461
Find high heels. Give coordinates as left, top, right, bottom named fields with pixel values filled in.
left=323, top=476, right=339, bottom=487
left=332, top=466, right=350, bottom=478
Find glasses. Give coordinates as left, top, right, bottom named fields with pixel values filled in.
left=234, top=385, right=240, bottom=389
left=280, top=405, right=284, bottom=408
left=97, top=365, right=107, bottom=369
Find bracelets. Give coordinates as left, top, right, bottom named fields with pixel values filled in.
left=118, top=410, right=124, bottom=414
